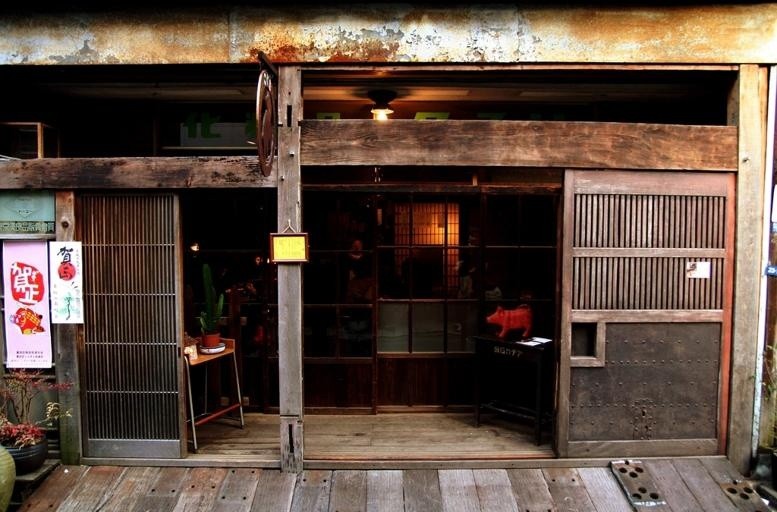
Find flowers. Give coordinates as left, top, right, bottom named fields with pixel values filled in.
left=0, top=358, right=77, bottom=445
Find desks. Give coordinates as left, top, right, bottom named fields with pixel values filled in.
left=471, top=327, right=553, bottom=447
left=181, top=338, right=248, bottom=451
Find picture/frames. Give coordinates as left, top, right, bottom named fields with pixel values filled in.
left=269, top=233, right=310, bottom=263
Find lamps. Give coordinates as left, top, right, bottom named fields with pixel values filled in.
left=366, top=87, right=399, bottom=114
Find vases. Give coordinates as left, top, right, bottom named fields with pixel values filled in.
left=5, top=435, right=48, bottom=474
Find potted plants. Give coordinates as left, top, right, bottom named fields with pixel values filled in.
left=191, top=262, right=228, bottom=347
